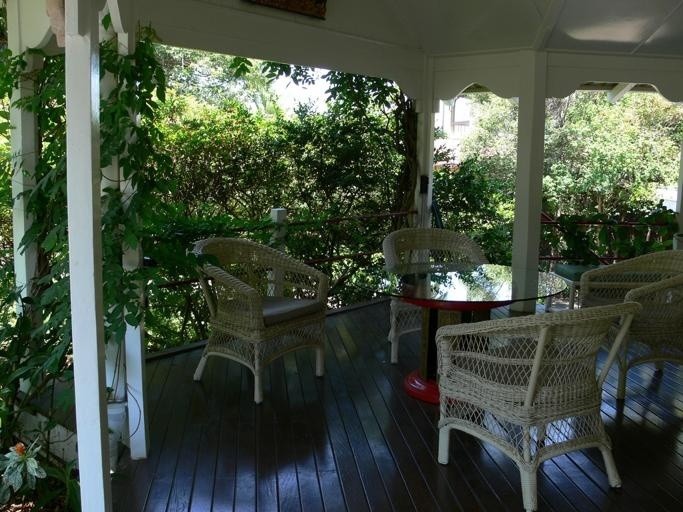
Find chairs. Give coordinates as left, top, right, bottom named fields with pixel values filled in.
left=382, top=228, right=490, bottom=364
left=578, top=250, right=683, bottom=399
left=193, top=238, right=330, bottom=404
left=435, top=302, right=643, bottom=512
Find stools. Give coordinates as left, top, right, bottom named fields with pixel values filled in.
left=544, top=263, right=599, bottom=312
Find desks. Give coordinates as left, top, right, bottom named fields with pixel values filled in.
left=351, top=264, right=567, bottom=404
